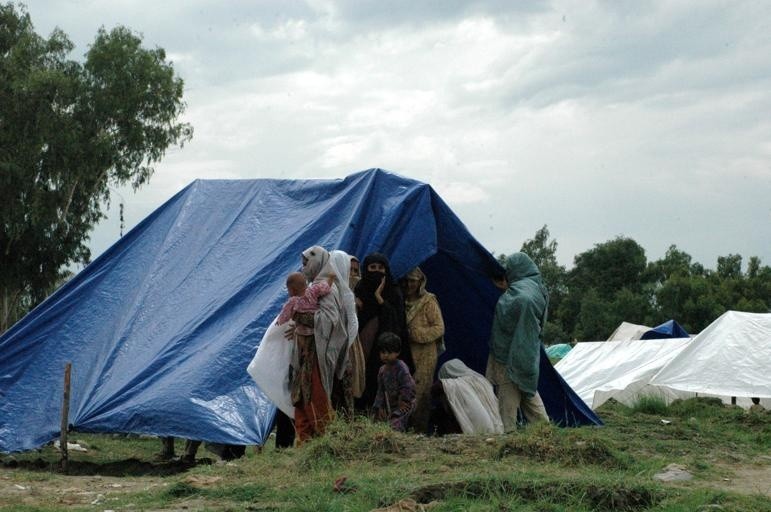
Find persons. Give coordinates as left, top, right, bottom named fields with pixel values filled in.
left=245, top=244, right=504, bottom=453
left=486, top=252, right=550, bottom=433
left=153, top=434, right=202, bottom=472
